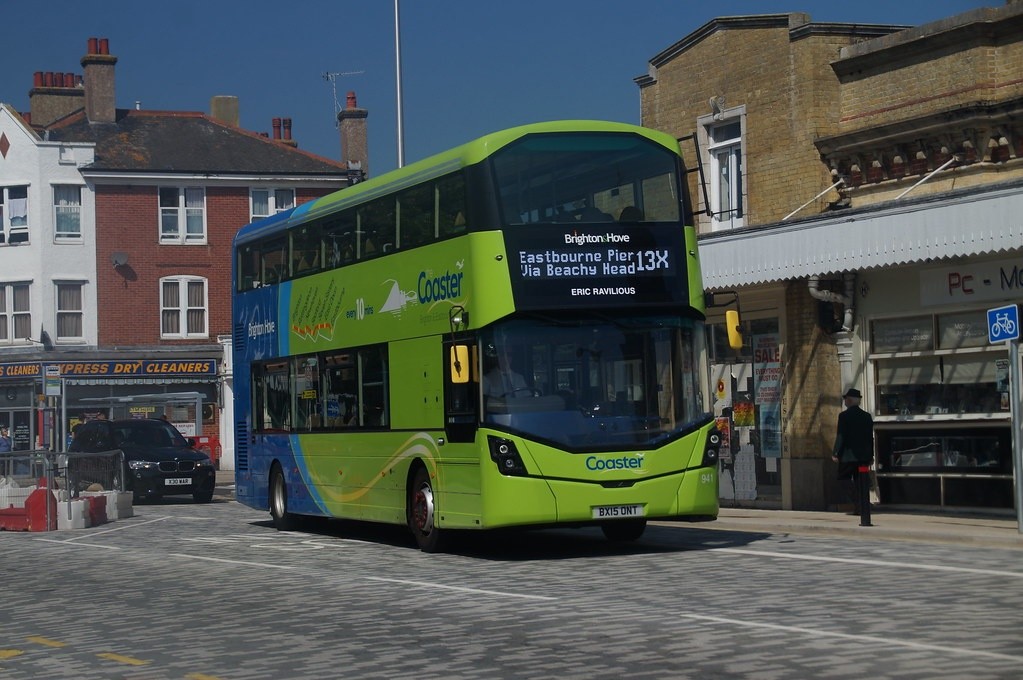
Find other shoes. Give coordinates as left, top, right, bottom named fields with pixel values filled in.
left=855, top=511, right=862, bottom=516
left=845, top=503, right=856, bottom=514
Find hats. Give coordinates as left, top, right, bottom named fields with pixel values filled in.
left=842, top=389, right=863, bottom=399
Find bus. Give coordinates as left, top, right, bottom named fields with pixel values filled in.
left=231, top=120, right=746, bottom=554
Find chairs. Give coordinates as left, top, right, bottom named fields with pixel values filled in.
left=238, top=231, right=388, bottom=285
left=275, top=400, right=385, bottom=429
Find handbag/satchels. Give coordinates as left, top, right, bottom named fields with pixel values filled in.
left=868, top=466, right=880, bottom=503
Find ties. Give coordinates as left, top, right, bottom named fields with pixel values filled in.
left=502, top=373, right=516, bottom=398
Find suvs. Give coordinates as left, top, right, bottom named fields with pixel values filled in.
left=65, top=418, right=216, bottom=507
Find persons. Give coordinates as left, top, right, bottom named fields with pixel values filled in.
left=72, top=409, right=108, bottom=440
left=0, top=428, right=12, bottom=474
left=832, top=389, right=875, bottom=516
left=482, top=347, right=533, bottom=400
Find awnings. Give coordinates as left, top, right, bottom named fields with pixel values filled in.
left=696, top=156, right=1023, bottom=293
